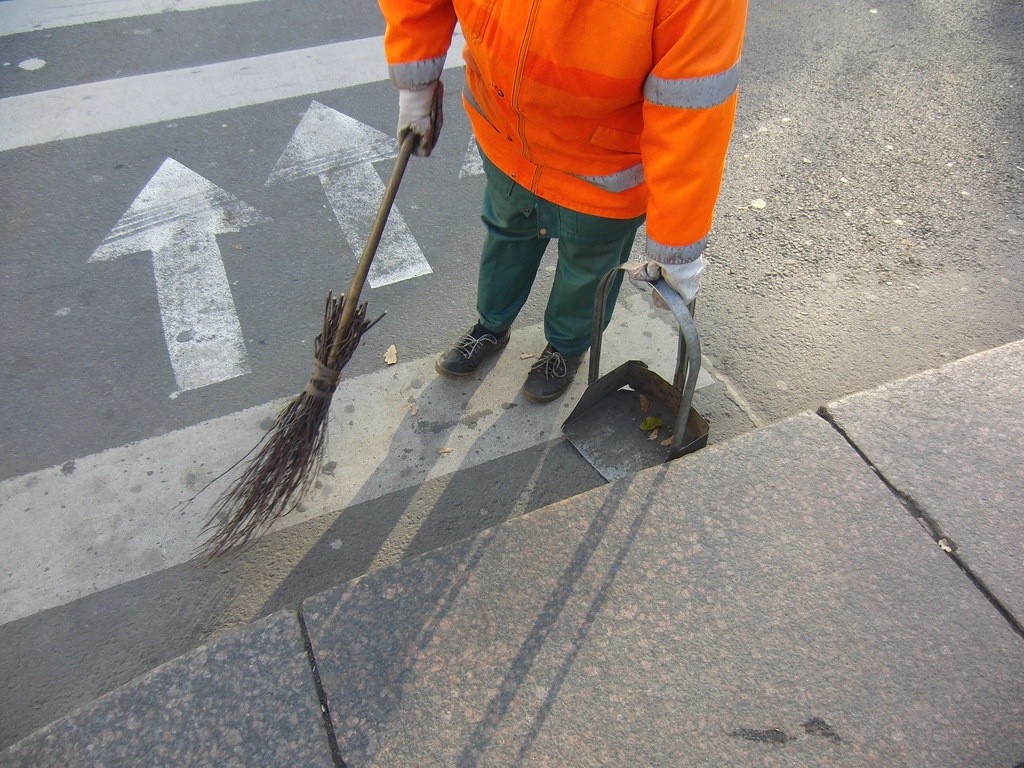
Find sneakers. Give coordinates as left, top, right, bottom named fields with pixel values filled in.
left=522, top=342, right=588, bottom=402
left=436, top=319, right=511, bottom=375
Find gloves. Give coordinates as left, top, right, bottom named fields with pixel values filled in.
left=397, top=80, right=444, bottom=157
left=646, top=253, right=704, bottom=310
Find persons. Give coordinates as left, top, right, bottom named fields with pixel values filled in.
left=378, top=1, right=748, bottom=402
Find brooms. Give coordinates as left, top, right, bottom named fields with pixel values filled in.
left=164, top=92, right=435, bottom=572
